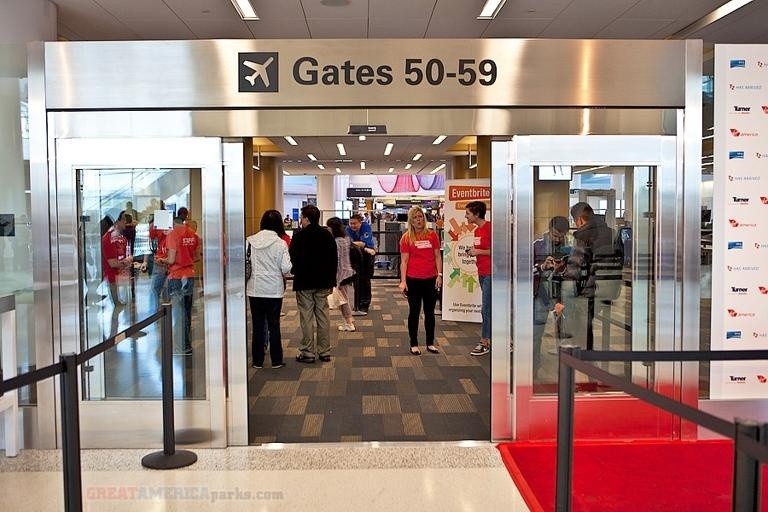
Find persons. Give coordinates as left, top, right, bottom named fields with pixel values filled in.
left=284, top=214, right=294, bottom=229
left=244, top=209, right=293, bottom=369
left=363, top=211, right=444, bottom=223
left=533, top=215, right=575, bottom=389
left=345, top=214, right=376, bottom=278
left=327, top=217, right=356, bottom=332
left=280, top=234, right=291, bottom=251
left=463, top=201, right=491, bottom=356
left=399, top=206, right=443, bottom=355
left=554, top=201, right=625, bottom=391
left=98, top=196, right=202, bottom=357
left=347, top=241, right=373, bottom=316
left=289, top=204, right=339, bottom=363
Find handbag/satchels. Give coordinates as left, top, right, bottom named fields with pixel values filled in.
left=246, top=260, right=251, bottom=279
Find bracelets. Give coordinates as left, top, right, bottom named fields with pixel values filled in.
left=435, top=273, right=443, bottom=278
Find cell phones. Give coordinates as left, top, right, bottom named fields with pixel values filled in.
left=551, top=259, right=560, bottom=271
left=402, top=290, right=408, bottom=298
left=465, top=250, right=474, bottom=257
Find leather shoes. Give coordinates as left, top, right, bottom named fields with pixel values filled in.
left=427, top=346, right=438, bottom=353
left=410, top=347, right=420, bottom=355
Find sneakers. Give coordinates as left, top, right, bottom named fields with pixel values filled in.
left=338, top=323, right=355, bottom=331
left=296, top=353, right=330, bottom=363
left=252, top=363, right=263, bottom=369
left=470, top=342, right=490, bottom=355
left=272, top=361, right=285, bottom=368
left=174, top=341, right=193, bottom=355
